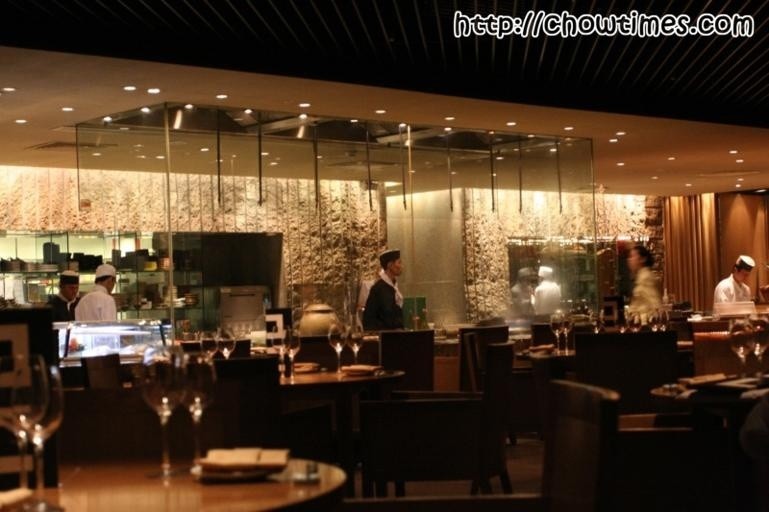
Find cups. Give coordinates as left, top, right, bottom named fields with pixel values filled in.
left=427, top=322, right=435, bottom=329
left=251, top=315, right=267, bottom=353
left=435, top=328, right=447, bottom=340
left=586, top=308, right=604, bottom=334
left=623, top=305, right=669, bottom=333
left=69, top=262, right=79, bottom=271
left=184, top=293, right=199, bottom=306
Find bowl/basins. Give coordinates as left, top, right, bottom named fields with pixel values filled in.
left=144, top=258, right=174, bottom=271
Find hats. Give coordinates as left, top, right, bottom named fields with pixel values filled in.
left=94, top=263, right=117, bottom=279
left=538, top=266, right=554, bottom=279
left=518, top=267, right=536, bottom=279
left=735, top=255, right=756, bottom=271
left=60, top=270, right=79, bottom=284
left=377, top=249, right=403, bottom=266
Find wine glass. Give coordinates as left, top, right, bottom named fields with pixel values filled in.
left=550, top=315, right=574, bottom=356
left=729, top=321, right=755, bottom=379
left=0, top=354, right=64, bottom=512
left=270, top=325, right=301, bottom=384
left=139, top=341, right=217, bottom=488
left=327, top=322, right=349, bottom=374
left=346, top=324, right=364, bottom=367
left=199, top=324, right=235, bottom=360
left=748, top=318, right=768, bottom=378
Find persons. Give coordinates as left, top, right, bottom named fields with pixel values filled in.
left=711, top=253, right=756, bottom=303
left=525, top=265, right=563, bottom=316
left=626, top=244, right=662, bottom=313
left=510, top=265, right=535, bottom=317
left=73, top=263, right=118, bottom=323
left=45, top=269, right=81, bottom=322
left=362, top=249, right=405, bottom=331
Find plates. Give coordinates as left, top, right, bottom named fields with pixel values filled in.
left=112, top=292, right=129, bottom=309
left=3, top=261, right=59, bottom=272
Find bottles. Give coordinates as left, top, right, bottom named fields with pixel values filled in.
left=663, top=288, right=669, bottom=305
